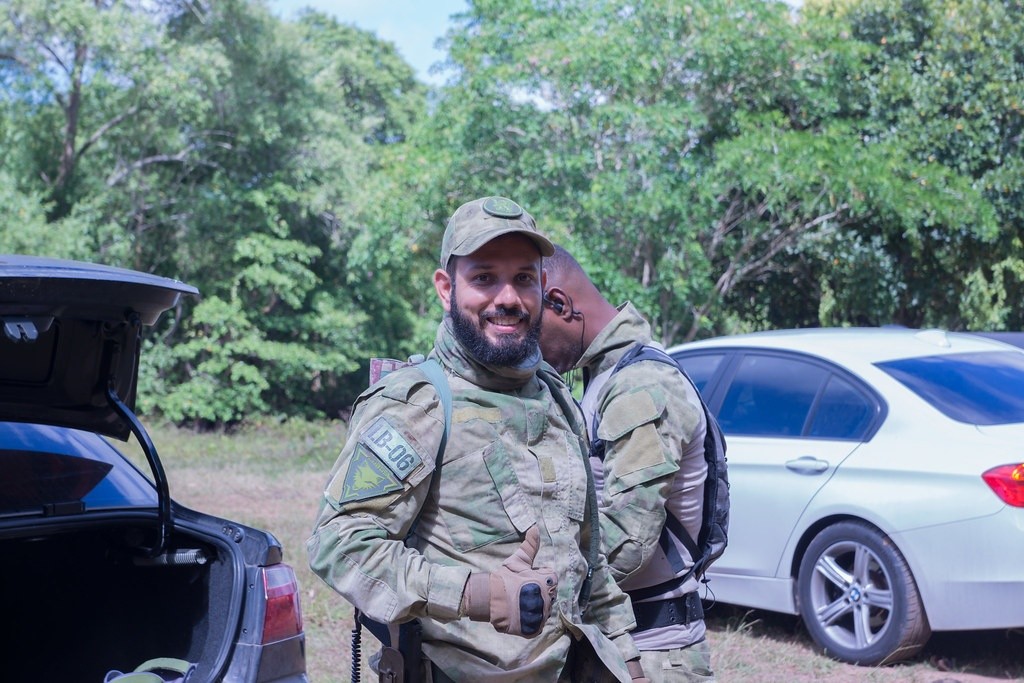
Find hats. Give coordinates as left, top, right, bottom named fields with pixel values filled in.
left=442, top=195, right=554, bottom=275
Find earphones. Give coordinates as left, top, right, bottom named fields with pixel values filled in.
left=544, top=297, right=562, bottom=312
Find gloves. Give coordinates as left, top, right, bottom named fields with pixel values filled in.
left=467, top=526, right=557, bottom=640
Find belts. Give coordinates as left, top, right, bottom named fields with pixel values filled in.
left=627, top=592, right=706, bottom=634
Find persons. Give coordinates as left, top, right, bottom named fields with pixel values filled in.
left=539, top=244, right=732, bottom=683
left=307, top=199, right=651, bottom=683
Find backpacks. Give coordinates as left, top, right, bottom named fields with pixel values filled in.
left=588, top=343, right=728, bottom=580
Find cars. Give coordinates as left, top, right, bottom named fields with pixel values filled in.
left=0, top=252, right=309, bottom=683
left=666, top=327, right=1024, bottom=666
